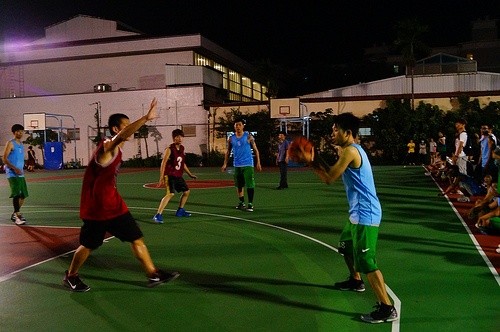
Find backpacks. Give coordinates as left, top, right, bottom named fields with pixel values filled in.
left=458, top=130, right=473, bottom=154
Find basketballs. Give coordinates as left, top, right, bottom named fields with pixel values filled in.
left=287, top=137, right=313, bottom=160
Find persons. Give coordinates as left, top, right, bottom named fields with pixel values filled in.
left=428, top=119, right=500, bottom=253
left=290, top=113, right=398, bottom=323
left=66, top=158, right=78, bottom=169
left=276, top=132, right=289, bottom=190
left=27, top=146, right=37, bottom=172
left=4, top=124, right=28, bottom=225
left=407, top=140, right=416, bottom=166
left=221, top=118, right=261, bottom=211
left=419, top=140, right=427, bottom=166
left=63, top=98, right=179, bottom=292
left=0, top=158, right=5, bottom=174
left=153, top=129, right=197, bottom=223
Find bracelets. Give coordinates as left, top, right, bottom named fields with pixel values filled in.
left=11, top=167, right=15, bottom=170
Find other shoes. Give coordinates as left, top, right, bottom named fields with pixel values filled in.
left=424, top=172, right=431, bottom=176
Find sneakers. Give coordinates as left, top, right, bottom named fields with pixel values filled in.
left=361, top=301, right=398, bottom=324
left=11, top=213, right=26, bottom=222
left=236, top=201, right=246, bottom=208
left=61, top=270, right=91, bottom=292
left=147, top=270, right=180, bottom=287
left=335, top=275, right=366, bottom=292
left=153, top=212, right=164, bottom=224
left=457, top=195, right=470, bottom=202
left=15, top=216, right=26, bottom=224
left=246, top=203, right=253, bottom=211
left=176, top=208, right=191, bottom=217
